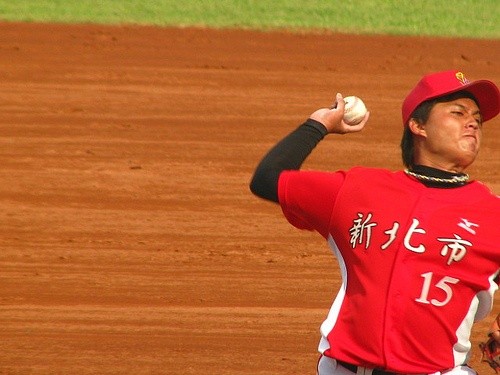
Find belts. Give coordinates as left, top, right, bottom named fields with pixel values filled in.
left=336, top=360, right=394, bottom=375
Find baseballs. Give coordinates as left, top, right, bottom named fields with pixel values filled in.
left=339, top=95, right=367, bottom=126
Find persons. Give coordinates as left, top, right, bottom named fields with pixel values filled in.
left=248, top=68, right=500, bottom=373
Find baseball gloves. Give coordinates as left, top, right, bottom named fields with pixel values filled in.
left=479, top=313, right=500, bottom=375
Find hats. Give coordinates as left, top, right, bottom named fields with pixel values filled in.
left=401, top=70, right=500, bottom=131
